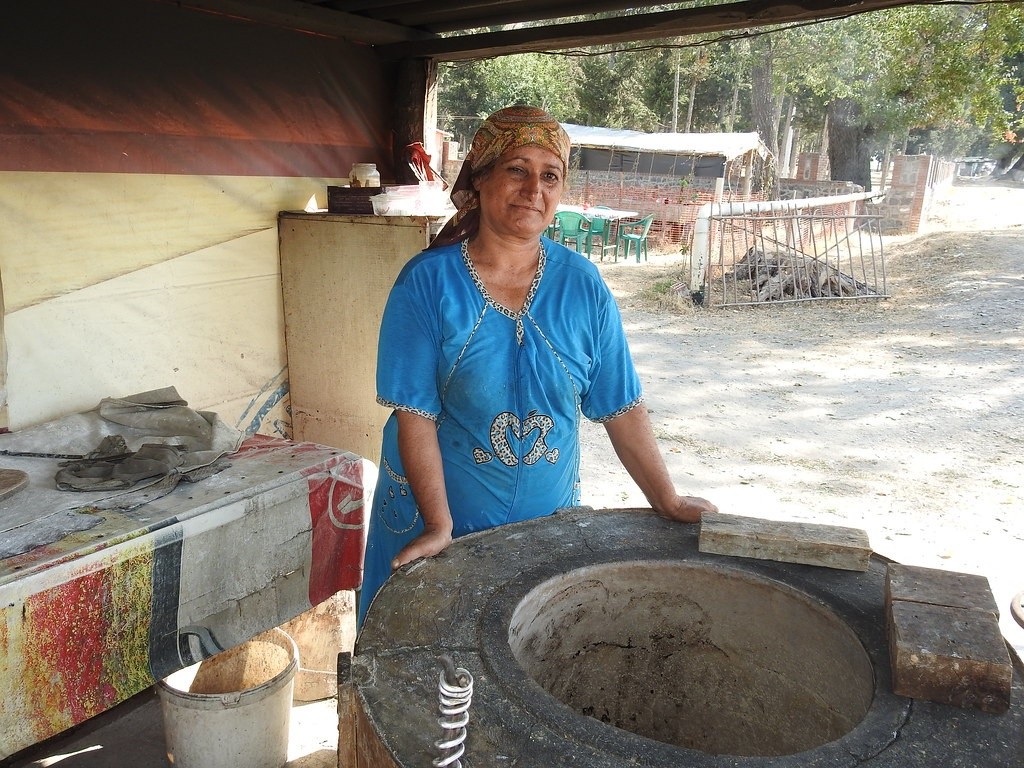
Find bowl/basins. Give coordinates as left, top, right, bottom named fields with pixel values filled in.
left=368, top=194, right=422, bottom=216
left=386, top=185, right=432, bottom=195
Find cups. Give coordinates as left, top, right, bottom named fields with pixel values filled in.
left=419, top=180, right=443, bottom=216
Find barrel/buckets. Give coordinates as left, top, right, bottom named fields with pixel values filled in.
left=275, top=590, right=356, bottom=701
left=156, top=628, right=300, bottom=768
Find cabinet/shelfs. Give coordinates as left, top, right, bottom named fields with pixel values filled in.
left=280, top=210, right=446, bottom=468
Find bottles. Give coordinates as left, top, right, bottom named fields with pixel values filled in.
left=580, top=193, right=584, bottom=204
left=583, top=200, right=589, bottom=210
left=349, top=162, right=381, bottom=187
left=588, top=193, right=594, bottom=206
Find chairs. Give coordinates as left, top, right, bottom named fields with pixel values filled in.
left=582, top=206, right=613, bottom=256
left=615, top=214, right=655, bottom=262
left=543, top=211, right=594, bottom=260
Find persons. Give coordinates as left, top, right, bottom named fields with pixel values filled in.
left=357, top=106, right=718, bottom=636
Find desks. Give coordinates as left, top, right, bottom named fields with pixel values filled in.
left=1, top=436, right=380, bottom=763
left=553, top=204, right=638, bottom=259
